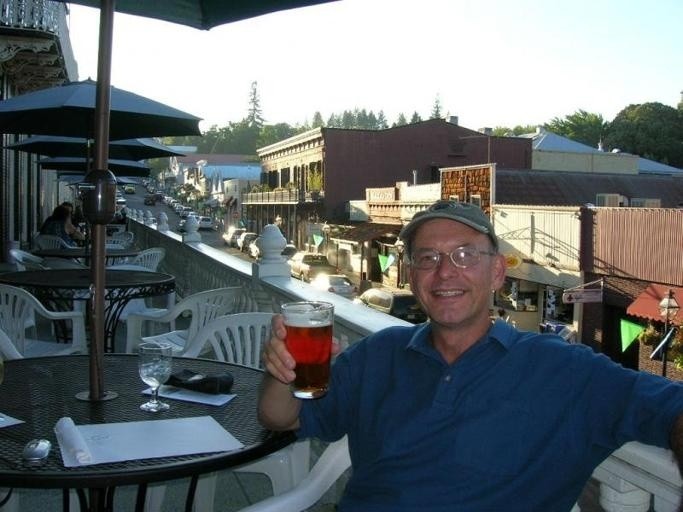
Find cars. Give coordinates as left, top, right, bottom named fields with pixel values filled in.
left=122, top=184, right=126, bottom=188
left=141, top=179, right=197, bottom=233
left=309, top=272, right=358, bottom=299
left=248, top=237, right=262, bottom=259
left=236, top=232, right=257, bottom=252
left=115, top=189, right=127, bottom=208
left=281, top=244, right=298, bottom=262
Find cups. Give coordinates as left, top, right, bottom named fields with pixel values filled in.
left=280, top=300, right=334, bottom=399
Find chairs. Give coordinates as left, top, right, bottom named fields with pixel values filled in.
left=0, top=220, right=578, bottom=512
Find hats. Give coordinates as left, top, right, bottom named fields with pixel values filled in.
left=398, top=201, right=498, bottom=245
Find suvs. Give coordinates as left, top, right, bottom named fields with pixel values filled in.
left=221, top=226, right=247, bottom=247
left=124, top=183, right=136, bottom=194
left=196, top=216, right=214, bottom=230
left=353, top=287, right=428, bottom=326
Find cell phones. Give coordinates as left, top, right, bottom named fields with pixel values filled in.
left=23, top=438, right=51, bottom=467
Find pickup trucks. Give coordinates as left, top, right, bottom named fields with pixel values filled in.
left=286, top=251, right=340, bottom=283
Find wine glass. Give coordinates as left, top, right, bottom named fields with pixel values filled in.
left=137, top=342, right=174, bottom=413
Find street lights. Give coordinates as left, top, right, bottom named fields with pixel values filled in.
left=656, top=288, right=680, bottom=378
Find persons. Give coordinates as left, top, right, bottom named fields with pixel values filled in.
left=257, top=200, right=682, bottom=512
left=498, top=309, right=509, bottom=320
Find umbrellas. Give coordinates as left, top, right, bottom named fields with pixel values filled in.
left=1, top=77, right=202, bottom=264
left=39, top=0, right=339, bottom=400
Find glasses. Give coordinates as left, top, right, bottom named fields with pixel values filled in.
left=405, top=248, right=496, bottom=271
